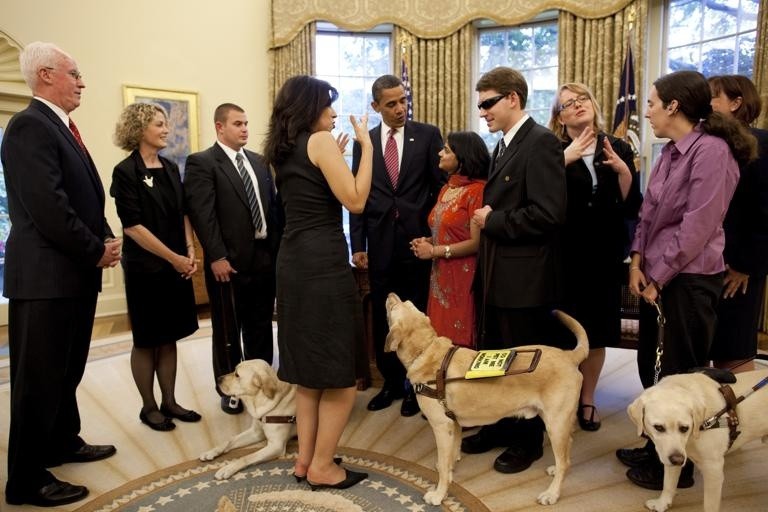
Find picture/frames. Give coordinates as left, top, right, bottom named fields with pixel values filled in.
left=119, top=82, right=202, bottom=187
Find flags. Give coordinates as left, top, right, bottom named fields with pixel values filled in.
left=612, top=39, right=641, bottom=175
left=399, top=48, right=414, bottom=121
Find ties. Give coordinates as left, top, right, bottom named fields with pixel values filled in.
left=382, top=128, right=404, bottom=217
left=67, top=117, right=93, bottom=180
left=231, top=153, right=264, bottom=232
left=494, top=138, right=508, bottom=175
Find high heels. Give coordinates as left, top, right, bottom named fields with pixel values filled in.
left=297, top=454, right=368, bottom=492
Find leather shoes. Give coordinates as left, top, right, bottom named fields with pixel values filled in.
left=217, top=391, right=244, bottom=415
left=137, top=398, right=203, bottom=434
left=43, top=434, right=116, bottom=470
left=459, top=414, right=544, bottom=474
left=4, top=472, right=89, bottom=506
left=367, top=376, right=423, bottom=415
left=577, top=395, right=601, bottom=432
left=618, top=423, right=696, bottom=490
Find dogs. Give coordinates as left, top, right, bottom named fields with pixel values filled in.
left=626, top=367, right=768, bottom=512
left=384, top=291, right=591, bottom=507
left=198, top=358, right=301, bottom=482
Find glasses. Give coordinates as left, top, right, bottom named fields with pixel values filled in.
left=558, top=91, right=590, bottom=111
left=41, top=64, right=82, bottom=81
left=477, top=91, right=508, bottom=109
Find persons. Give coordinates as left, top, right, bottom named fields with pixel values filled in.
left=109, top=104, right=203, bottom=433
left=256, top=69, right=768, bottom=492
left=182, top=102, right=282, bottom=415
left=0, top=41, right=127, bottom=507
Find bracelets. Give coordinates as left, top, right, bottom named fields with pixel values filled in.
left=187, top=245, right=195, bottom=249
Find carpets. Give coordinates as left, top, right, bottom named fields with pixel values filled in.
left=66, top=437, right=496, bottom=512
